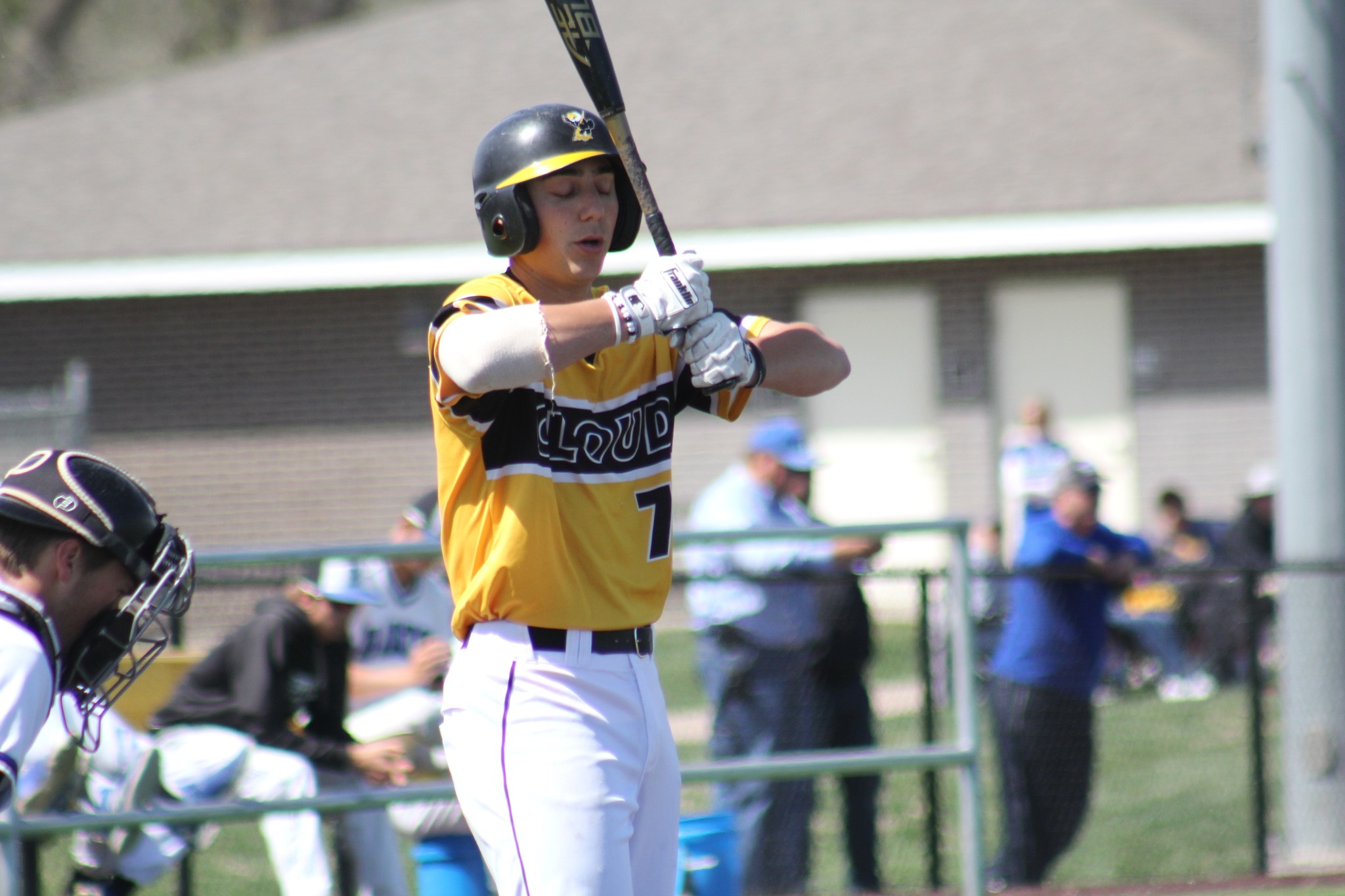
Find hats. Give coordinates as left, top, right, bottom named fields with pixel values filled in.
left=300, top=556, right=385, bottom=608
left=1058, top=459, right=1112, bottom=485
left=745, top=420, right=818, bottom=474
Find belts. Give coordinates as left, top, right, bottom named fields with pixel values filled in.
left=461, top=622, right=655, bottom=659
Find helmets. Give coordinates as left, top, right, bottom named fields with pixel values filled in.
left=472, top=103, right=648, bottom=258
left=1, top=447, right=159, bottom=573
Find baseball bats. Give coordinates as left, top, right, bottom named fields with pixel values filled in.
left=544, top=1, right=735, bottom=396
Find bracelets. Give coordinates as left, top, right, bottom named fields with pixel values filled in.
left=601, top=291, right=636, bottom=346
left=744, top=338, right=766, bottom=389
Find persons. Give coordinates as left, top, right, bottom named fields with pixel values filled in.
left=0, top=447, right=195, bottom=808
left=429, top=104, right=851, bottom=896
left=0, top=487, right=469, bottom=896
left=966, top=401, right=1282, bottom=887
left=684, top=417, right=886, bottom=896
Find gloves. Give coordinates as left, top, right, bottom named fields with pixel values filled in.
left=616, top=251, right=713, bottom=339
left=669, top=312, right=755, bottom=388
